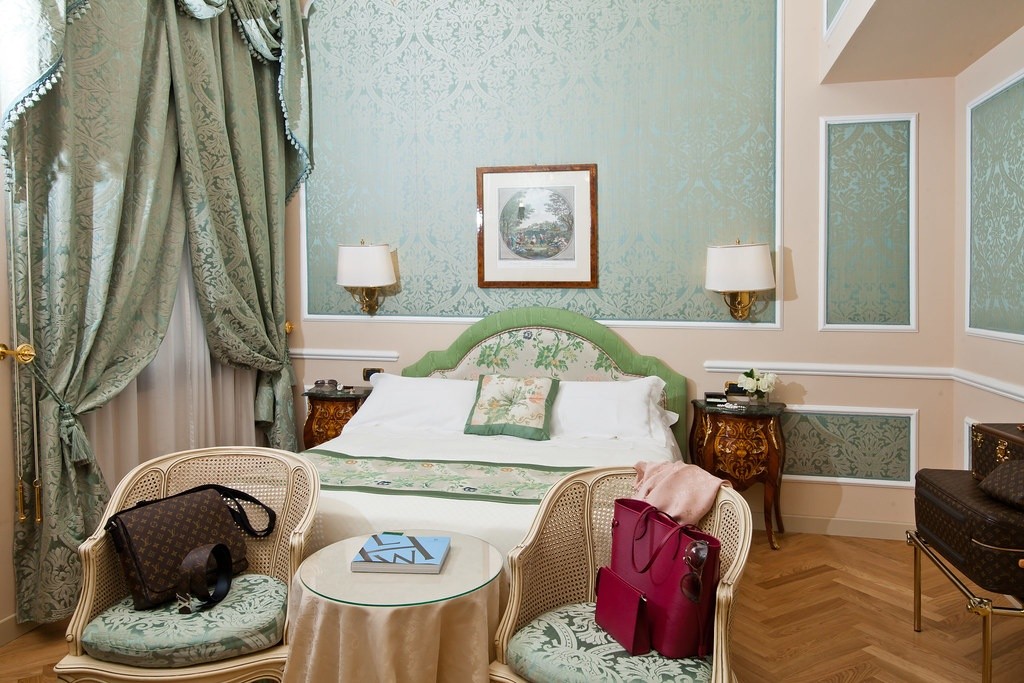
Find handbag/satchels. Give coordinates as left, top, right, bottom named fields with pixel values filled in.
left=595, top=565, right=649, bottom=655
left=610, top=498, right=722, bottom=659
left=105, top=489, right=248, bottom=610
left=978, top=460, right=1024, bottom=506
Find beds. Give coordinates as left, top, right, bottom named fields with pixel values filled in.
left=227, top=307, right=689, bottom=619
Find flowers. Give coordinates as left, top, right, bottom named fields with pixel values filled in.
left=737, top=368, right=779, bottom=399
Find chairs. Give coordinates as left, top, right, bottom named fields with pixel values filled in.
left=53, top=446, right=321, bottom=683
left=488, top=461, right=753, bottom=683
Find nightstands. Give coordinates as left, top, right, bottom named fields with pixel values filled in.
left=689, top=400, right=787, bottom=551
left=301, top=386, right=374, bottom=451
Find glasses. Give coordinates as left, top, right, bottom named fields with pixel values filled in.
left=681, top=540, right=708, bottom=605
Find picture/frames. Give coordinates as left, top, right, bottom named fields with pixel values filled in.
left=477, top=164, right=598, bottom=288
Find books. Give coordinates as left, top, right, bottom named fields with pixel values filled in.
left=350, top=535, right=452, bottom=573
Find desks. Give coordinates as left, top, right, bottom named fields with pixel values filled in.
left=906, top=530, right=1024, bottom=683
left=282, top=530, right=510, bottom=683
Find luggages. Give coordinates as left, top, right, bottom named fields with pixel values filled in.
left=915, top=468, right=1024, bottom=595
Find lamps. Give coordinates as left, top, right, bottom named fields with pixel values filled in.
left=337, top=239, right=397, bottom=311
left=705, top=238, right=776, bottom=321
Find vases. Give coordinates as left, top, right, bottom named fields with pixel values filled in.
left=748, top=391, right=770, bottom=406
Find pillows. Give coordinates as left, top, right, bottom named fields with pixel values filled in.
left=464, top=374, right=561, bottom=439
left=551, top=376, right=668, bottom=450
left=342, top=373, right=479, bottom=436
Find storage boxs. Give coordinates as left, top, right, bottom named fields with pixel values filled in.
left=972, top=423, right=1024, bottom=508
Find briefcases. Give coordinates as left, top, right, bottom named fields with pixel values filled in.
left=972, top=422, right=1023, bottom=484
